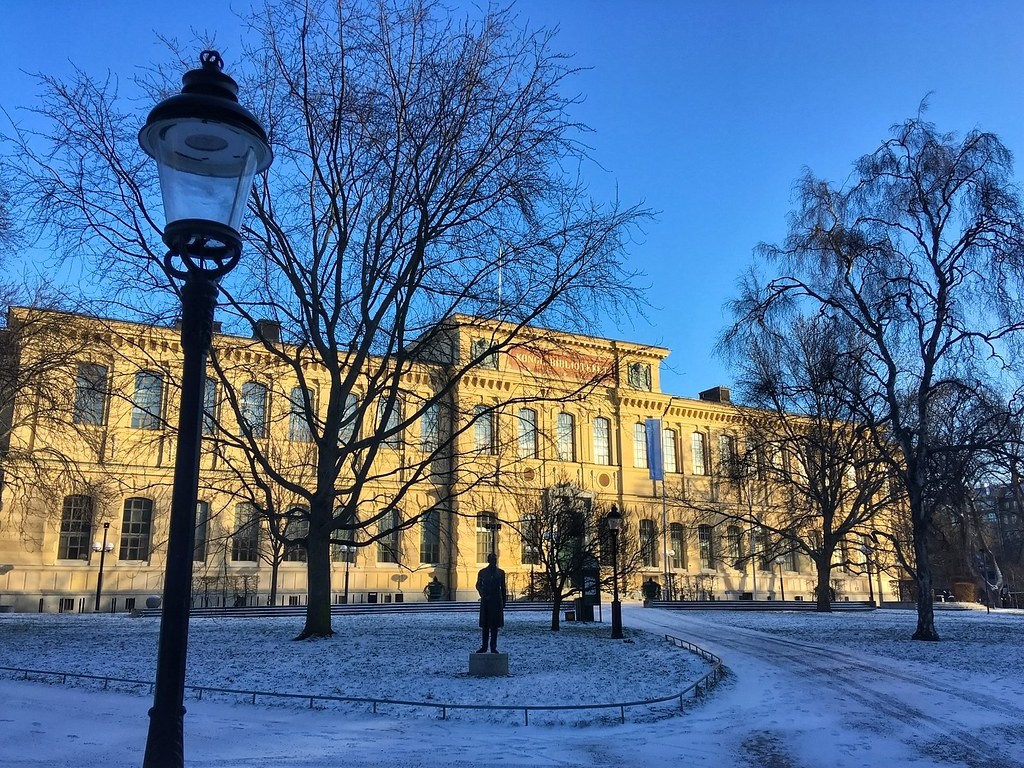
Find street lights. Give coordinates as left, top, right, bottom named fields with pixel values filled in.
left=525, top=544, right=539, bottom=600
left=91, top=541, right=114, bottom=614
left=133, top=41, right=276, bottom=768
left=340, top=545, right=356, bottom=604
left=662, top=550, right=675, bottom=601
left=861, top=544, right=876, bottom=606
left=775, top=556, right=785, bottom=601
left=606, top=503, right=623, bottom=640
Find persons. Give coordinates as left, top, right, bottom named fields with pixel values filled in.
left=476, top=554, right=506, bottom=654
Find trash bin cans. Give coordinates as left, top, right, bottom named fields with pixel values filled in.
left=743, top=593, right=753, bottom=600
left=368, top=592, right=377, bottom=603
left=576, top=597, right=594, bottom=621
